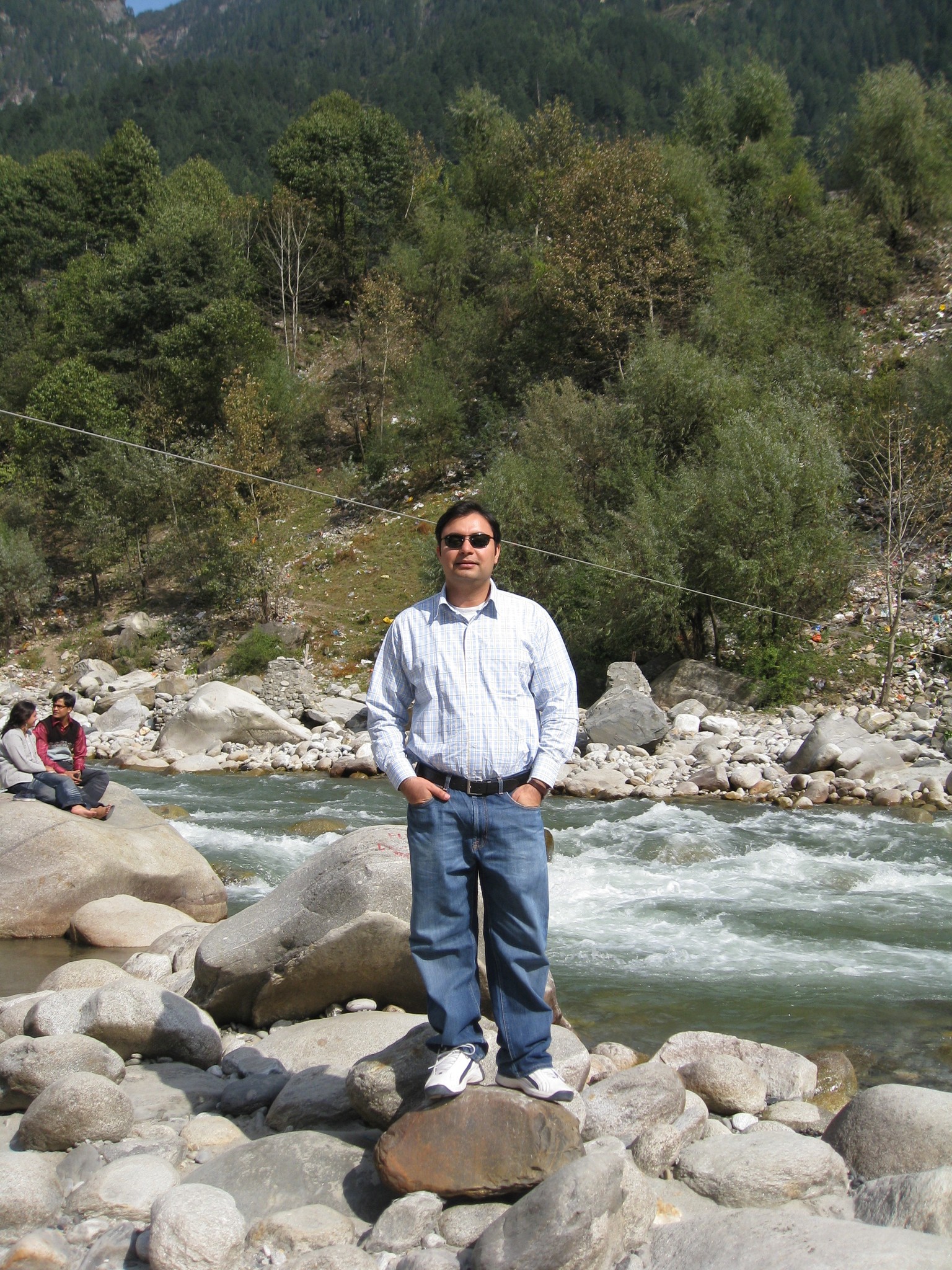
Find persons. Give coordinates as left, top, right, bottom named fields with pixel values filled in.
left=0, top=700, right=111, bottom=819
left=365, top=501, right=573, bottom=1102
left=32, top=692, right=115, bottom=821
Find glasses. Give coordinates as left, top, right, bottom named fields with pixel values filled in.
left=442, top=534, right=495, bottom=548
left=53, top=704, right=66, bottom=709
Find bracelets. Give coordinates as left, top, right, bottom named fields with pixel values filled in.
left=78, top=769, right=81, bottom=778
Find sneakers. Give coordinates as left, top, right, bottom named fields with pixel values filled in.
left=495, top=1066, right=575, bottom=1102
left=424, top=1043, right=484, bottom=1100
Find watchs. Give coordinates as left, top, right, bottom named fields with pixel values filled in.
left=526, top=777, right=552, bottom=799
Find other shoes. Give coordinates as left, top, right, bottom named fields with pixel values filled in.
left=12, top=793, right=37, bottom=802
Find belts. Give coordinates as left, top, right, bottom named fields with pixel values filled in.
left=415, top=762, right=530, bottom=797
